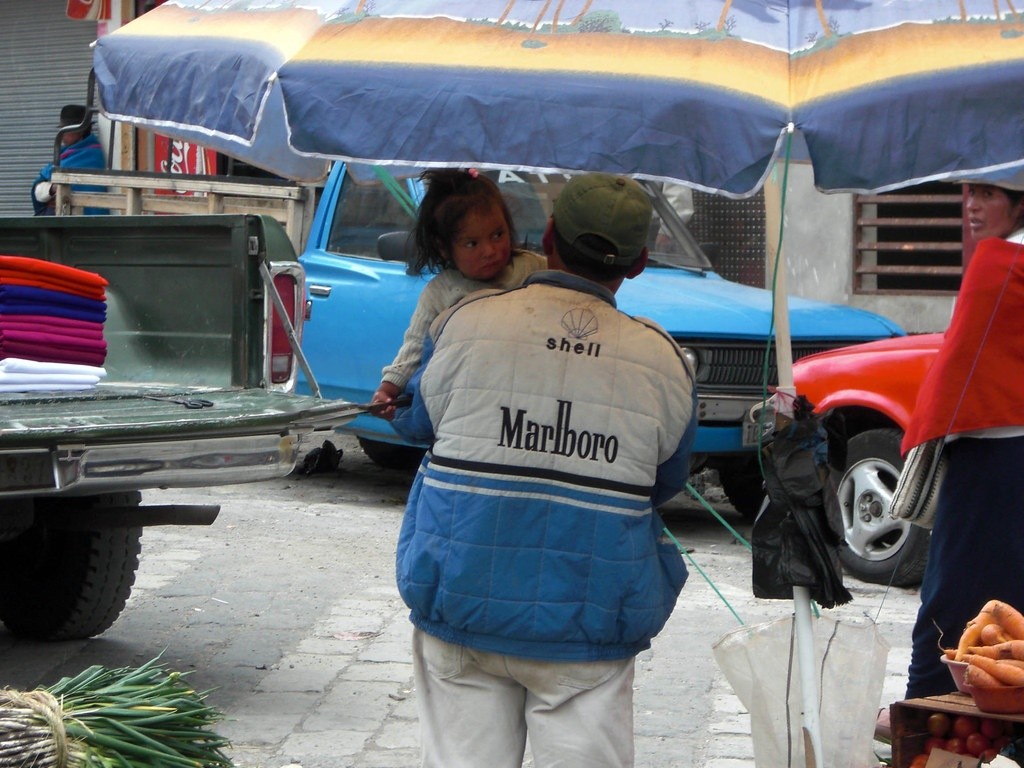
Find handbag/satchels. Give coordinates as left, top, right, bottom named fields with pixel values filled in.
left=889, top=434, right=947, bottom=530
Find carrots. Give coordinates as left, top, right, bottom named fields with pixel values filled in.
left=942, top=598, right=1024, bottom=688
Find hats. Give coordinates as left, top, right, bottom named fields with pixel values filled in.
left=553, top=171, right=653, bottom=266
left=56, top=104, right=97, bottom=128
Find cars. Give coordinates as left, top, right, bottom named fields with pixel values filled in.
left=794, top=326, right=959, bottom=593
left=291, top=162, right=911, bottom=518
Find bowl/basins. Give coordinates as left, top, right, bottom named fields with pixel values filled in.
left=939, top=655, right=977, bottom=693
left=968, top=685, right=1024, bottom=715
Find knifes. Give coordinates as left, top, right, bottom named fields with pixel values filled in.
left=288, top=392, right=415, bottom=424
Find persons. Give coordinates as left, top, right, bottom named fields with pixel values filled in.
left=873, top=166, right=1024, bottom=746
left=32, top=104, right=110, bottom=216
left=391, top=172, right=698, bottom=768
left=370, top=170, right=548, bottom=423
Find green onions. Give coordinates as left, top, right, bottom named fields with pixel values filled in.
left=0, top=644, right=240, bottom=768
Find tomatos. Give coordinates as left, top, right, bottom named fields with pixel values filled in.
left=923, top=712, right=1024, bottom=764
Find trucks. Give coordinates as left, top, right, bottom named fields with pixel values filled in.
left=1, top=215, right=372, bottom=647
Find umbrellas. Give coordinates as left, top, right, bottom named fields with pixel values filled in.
left=90, top=0, right=1024, bottom=768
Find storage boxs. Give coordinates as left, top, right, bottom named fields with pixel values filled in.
left=888, top=689, right=1024, bottom=768
